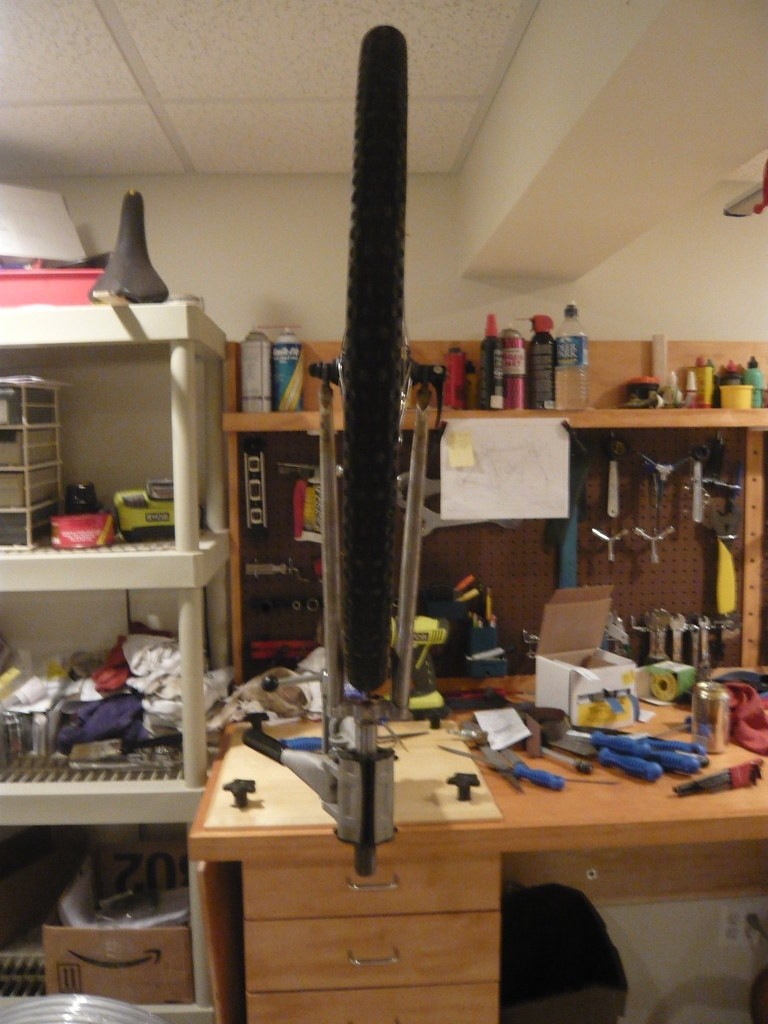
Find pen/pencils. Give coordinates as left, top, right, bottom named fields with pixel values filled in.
left=490, top=615, right=496, bottom=628
left=477, top=582, right=484, bottom=616
left=473, top=613, right=483, bottom=628
left=486, top=587, right=492, bottom=622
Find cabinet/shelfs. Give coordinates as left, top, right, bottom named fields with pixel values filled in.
left=0, top=302, right=227, bottom=1024
left=0, top=384, right=61, bottom=551
left=0, top=430, right=55, bottom=465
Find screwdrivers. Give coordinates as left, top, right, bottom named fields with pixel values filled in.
left=439, top=745, right=565, bottom=791
left=278, top=732, right=429, bottom=751
left=654, top=715, right=692, bottom=738
left=591, top=731, right=699, bottom=774
left=598, top=747, right=663, bottom=782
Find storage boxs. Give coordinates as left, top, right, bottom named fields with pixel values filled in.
left=0, top=269, right=106, bottom=306
left=0, top=505, right=57, bottom=544
left=0, top=388, right=51, bottom=425
left=44, top=839, right=193, bottom=1004
left=0, top=467, right=55, bottom=507
left=0, top=825, right=69, bottom=949
left=535, top=585, right=636, bottom=716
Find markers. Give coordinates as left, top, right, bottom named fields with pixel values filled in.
left=455, top=589, right=480, bottom=602
left=453, top=574, right=475, bottom=591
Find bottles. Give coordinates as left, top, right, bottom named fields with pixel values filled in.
left=443, top=345, right=465, bottom=408
left=528, top=315, right=555, bottom=409
left=242, top=325, right=271, bottom=412
left=478, top=314, right=503, bottom=409
left=556, top=304, right=588, bottom=409
left=742, top=356, right=763, bottom=407
left=502, top=328, right=525, bottom=408
left=272, top=330, right=303, bottom=412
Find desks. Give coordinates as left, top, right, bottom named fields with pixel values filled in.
left=188, top=668, right=768, bottom=1024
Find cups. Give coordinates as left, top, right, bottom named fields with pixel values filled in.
left=685, top=365, right=714, bottom=409
left=719, top=385, right=754, bottom=409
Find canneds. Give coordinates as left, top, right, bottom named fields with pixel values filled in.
left=691, top=682, right=729, bottom=753
